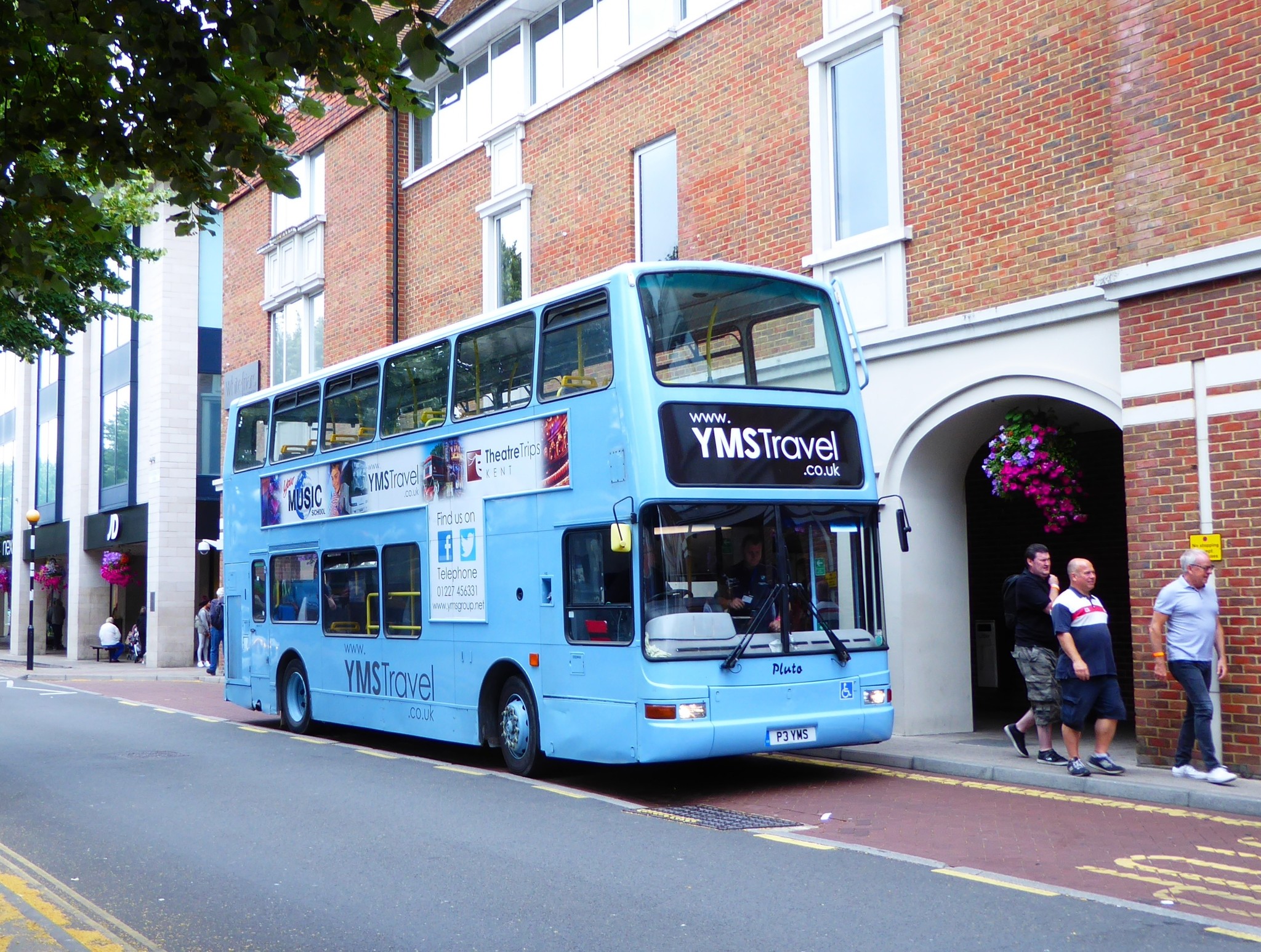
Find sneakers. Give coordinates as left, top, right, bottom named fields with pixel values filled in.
left=1004, top=724, right=1028, bottom=757
left=1036, top=748, right=1068, bottom=765
left=1173, top=765, right=1208, bottom=779
left=207, top=669, right=215, bottom=675
left=1067, top=757, right=1090, bottom=777
left=1086, top=751, right=1125, bottom=773
left=1206, top=768, right=1237, bottom=783
left=224, top=674, right=225, bottom=676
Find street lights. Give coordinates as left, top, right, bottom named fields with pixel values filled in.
left=26, top=509, right=41, bottom=670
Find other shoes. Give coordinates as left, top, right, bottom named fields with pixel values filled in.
left=197, top=660, right=204, bottom=667
left=1189, top=564, right=1215, bottom=571
left=204, top=661, right=211, bottom=667
left=111, top=659, right=120, bottom=662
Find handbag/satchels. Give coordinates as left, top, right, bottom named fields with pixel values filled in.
left=194, top=615, right=198, bottom=629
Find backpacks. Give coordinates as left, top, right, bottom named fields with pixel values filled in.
left=210, top=598, right=223, bottom=631
left=1001, top=568, right=1049, bottom=634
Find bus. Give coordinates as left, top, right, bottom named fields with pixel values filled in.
left=220, top=260, right=915, bottom=778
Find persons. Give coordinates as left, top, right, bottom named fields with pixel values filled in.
left=136, top=606, right=147, bottom=664
left=330, top=462, right=343, bottom=517
left=206, top=587, right=225, bottom=676
left=1150, top=549, right=1237, bottom=783
left=1003, top=544, right=1070, bottom=766
left=99, top=617, right=125, bottom=662
left=1051, top=558, right=1127, bottom=777
left=714, top=533, right=791, bottom=634
left=288, top=571, right=319, bottom=621
left=47, top=597, right=66, bottom=651
left=197, top=600, right=211, bottom=667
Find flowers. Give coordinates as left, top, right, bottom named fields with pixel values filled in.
left=101, top=551, right=142, bottom=588
left=982, top=405, right=1089, bottom=534
left=33, top=559, right=67, bottom=594
left=0, top=567, right=12, bottom=597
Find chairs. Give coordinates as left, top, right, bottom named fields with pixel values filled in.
left=279, top=411, right=447, bottom=460
left=556, top=376, right=598, bottom=398
left=330, top=622, right=361, bottom=634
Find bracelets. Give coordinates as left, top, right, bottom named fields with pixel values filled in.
left=776, top=617, right=780, bottom=621
left=1153, top=652, right=1164, bottom=657
left=1051, top=584, right=1060, bottom=590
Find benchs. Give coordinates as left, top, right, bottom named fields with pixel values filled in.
left=90, top=645, right=115, bottom=663
